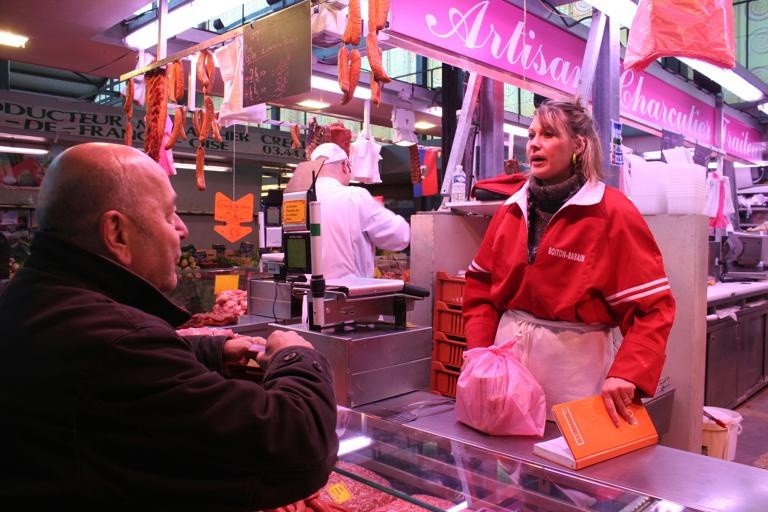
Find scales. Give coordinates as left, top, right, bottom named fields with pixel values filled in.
left=273, top=159, right=431, bottom=335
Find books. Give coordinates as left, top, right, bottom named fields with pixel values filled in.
left=532, top=392, right=659, bottom=471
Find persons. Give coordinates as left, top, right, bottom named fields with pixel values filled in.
left=0, top=141, right=341, bottom=511
left=309, top=142, right=410, bottom=283
left=458, top=94, right=678, bottom=432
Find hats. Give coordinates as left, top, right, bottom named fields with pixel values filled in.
left=310, top=143, right=348, bottom=165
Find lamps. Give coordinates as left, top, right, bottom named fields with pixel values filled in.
left=1, top=27, right=55, bottom=166
left=123, top=0, right=269, bottom=173
left=300, top=77, right=532, bottom=167
left=583, top=0, right=768, bottom=171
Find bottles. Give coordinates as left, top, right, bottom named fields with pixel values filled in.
left=451, top=164, right=466, bottom=203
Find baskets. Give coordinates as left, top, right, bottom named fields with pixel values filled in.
left=429, top=269, right=466, bottom=401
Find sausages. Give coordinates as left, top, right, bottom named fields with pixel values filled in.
left=335, top=1, right=391, bottom=108
left=263, top=492, right=352, bottom=512
left=123, top=50, right=224, bottom=193
left=289, top=124, right=302, bottom=150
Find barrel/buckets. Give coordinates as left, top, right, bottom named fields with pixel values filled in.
left=699, top=410, right=729, bottom=460
left=701, top=404, right=744, bottom=460
